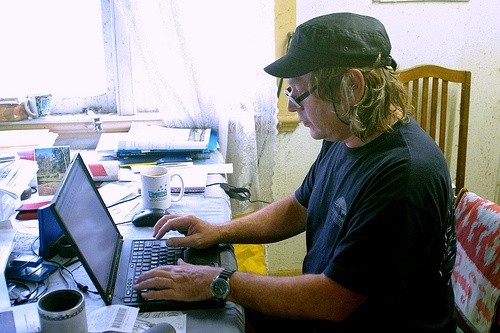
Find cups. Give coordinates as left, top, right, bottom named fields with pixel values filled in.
left=37, top=288, right=88, bottom=333
left=139, top=165, right=185, bottom=209
left=23, top=88, right=52, bottom=119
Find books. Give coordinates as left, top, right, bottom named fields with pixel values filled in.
left=138, top=165, right=207, bottom=193
left=96, top=121, right=220, bottom=182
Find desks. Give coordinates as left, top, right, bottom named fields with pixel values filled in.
left=6, top=137, right=245, bottom=333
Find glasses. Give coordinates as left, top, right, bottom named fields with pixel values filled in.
left=283, top=80, right=323, bottom=109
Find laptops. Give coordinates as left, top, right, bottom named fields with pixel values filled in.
left=49, top=153, right=227, bottom=313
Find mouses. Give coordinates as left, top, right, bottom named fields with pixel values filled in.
left=132, top=207, right=170, bottom=227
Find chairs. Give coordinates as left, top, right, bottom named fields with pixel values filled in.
left=398, top=64, right=500, bottom=333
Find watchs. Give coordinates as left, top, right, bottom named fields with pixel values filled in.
left=210, top=267, right=236, bottom=300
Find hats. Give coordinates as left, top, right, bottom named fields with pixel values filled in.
left=263, top=11, right=397, bottom=79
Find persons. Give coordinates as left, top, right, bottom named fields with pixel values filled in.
left=133, top=13, right=456, bottom=333
